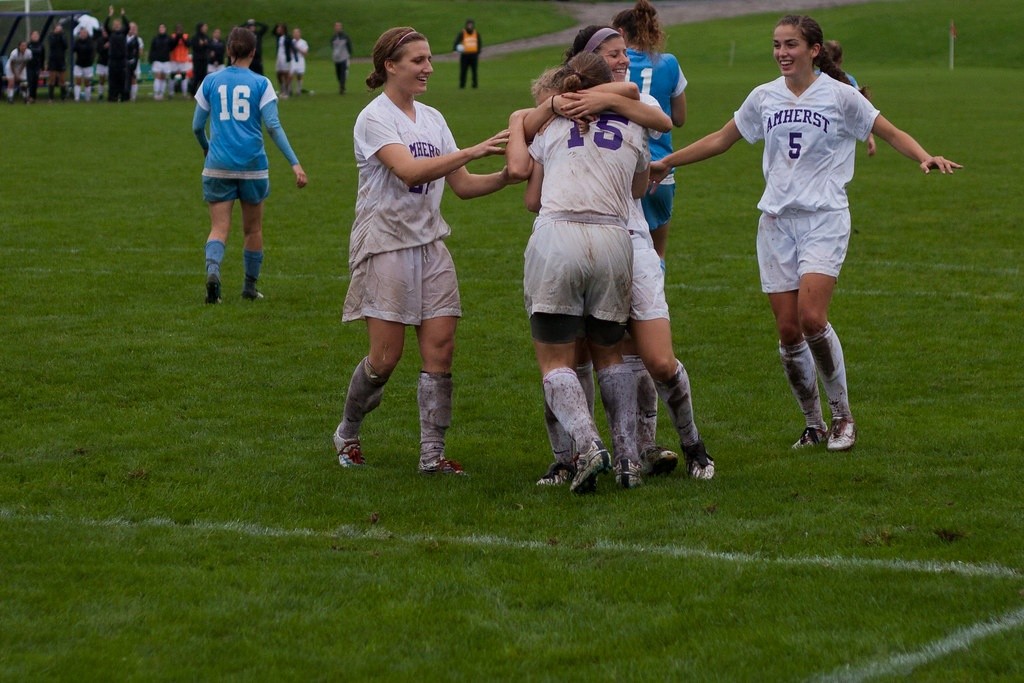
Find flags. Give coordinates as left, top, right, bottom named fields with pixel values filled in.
left=950, top=19, right=959, bottom=39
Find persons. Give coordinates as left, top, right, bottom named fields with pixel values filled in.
left=816, top=38, right=876, bottom=157
left=0, top=4, right=310, bottom=103
left=192, top=25, right=307, bottom=304
left=331, top=27, right=528, bottom=475
left=644, top=14, right=962, bottom=452
left=505, top=22, right=715, bottom=494
left=453, top=19, right=483, bottom=88
left=611, top=0, right=686, bottom=281
left=332, top=22, right=354, bottom=95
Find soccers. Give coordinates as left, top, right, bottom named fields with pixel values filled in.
left=456, top=44, right=465, bottom=53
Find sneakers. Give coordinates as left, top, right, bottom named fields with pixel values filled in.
left=686, top=456, right=715, bottom=481
left=613, top=457, right=642, bottom=488
left=827, top=416, right=856, bottom=451
left=332, top=422, right=366, bottom=467
left=205, top=274, right=222, bottom=304
left=242, top=289, right=264, bottom=300
left=570, top=440, right=612, bottom=496
left=417, top=458, right=465, bottom=476
left=645, top=449, right=679, bottom=476
left=792, top=421, right=828, bottom=448
left=536, top=460, right=576, bottom=488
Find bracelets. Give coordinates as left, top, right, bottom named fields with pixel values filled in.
left=551, top=94, right=556, bottom=112
